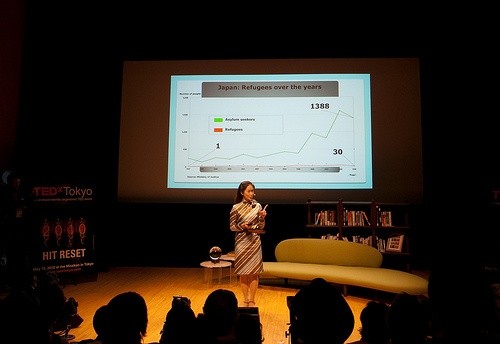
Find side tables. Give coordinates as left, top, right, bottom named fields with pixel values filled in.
left=200, top=254, right=235, bottom=285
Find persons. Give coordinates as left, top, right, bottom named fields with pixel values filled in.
left=229, top=180, right=268, bottom=309
left=79, top=278, right=435, bottom=344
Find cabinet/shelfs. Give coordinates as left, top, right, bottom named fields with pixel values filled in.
left=305, top=199, right=411, bottom=272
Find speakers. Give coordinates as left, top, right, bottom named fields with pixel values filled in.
left=238, top=307, right=262, bottom=344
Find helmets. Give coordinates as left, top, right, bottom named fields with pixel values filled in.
left=209, top=246, right=222, bottom=261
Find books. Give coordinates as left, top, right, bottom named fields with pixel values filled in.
left=314, top=210, right=404, bottom=252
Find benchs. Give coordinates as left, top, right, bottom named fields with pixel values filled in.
left=258, top=238, right=429, bottom=297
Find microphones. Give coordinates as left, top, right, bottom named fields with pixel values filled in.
left=252, top=204, right=256, bottom=208
